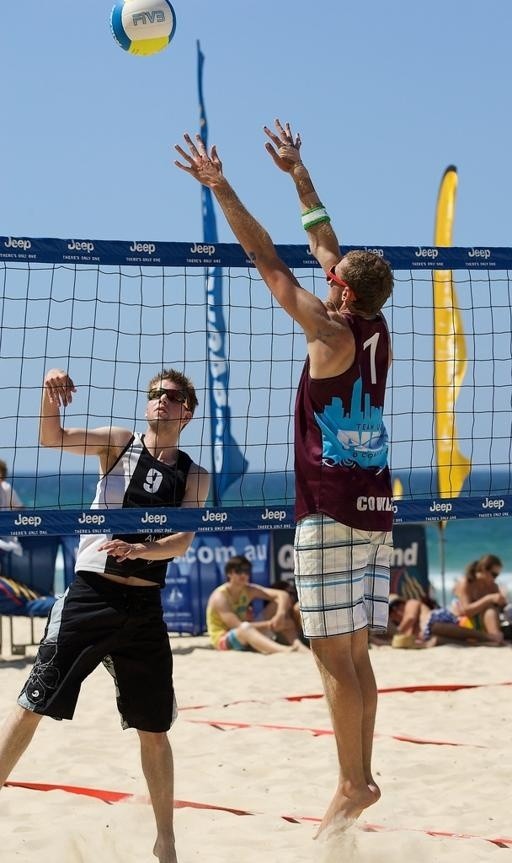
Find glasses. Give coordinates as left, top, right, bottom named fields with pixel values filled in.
left=234, top=567, right=250, bottom=574
left=326, top=265, right=355, bottom=299
left=488, top=570, right=499, bottom=579
left=148, top=388, right=187, bottom=405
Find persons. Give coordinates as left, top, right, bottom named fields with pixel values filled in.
left=0, top=458, right=27, bottom=556
left=205, top=551, right=312, bottom=653
left=0, top=364, right=215, bottom=862
left=383, top=587, right=505, bottom=647
left=173, top=112, right=400, bottom=848
left=447, top=552, right=512, bottom=643
left=262, top=580, right=305, bottom=638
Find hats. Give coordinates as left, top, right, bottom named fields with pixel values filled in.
left=387, top=594, right=404, bottom=606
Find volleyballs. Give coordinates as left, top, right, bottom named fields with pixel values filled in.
left=110, top=0, right=176, bottom=57
